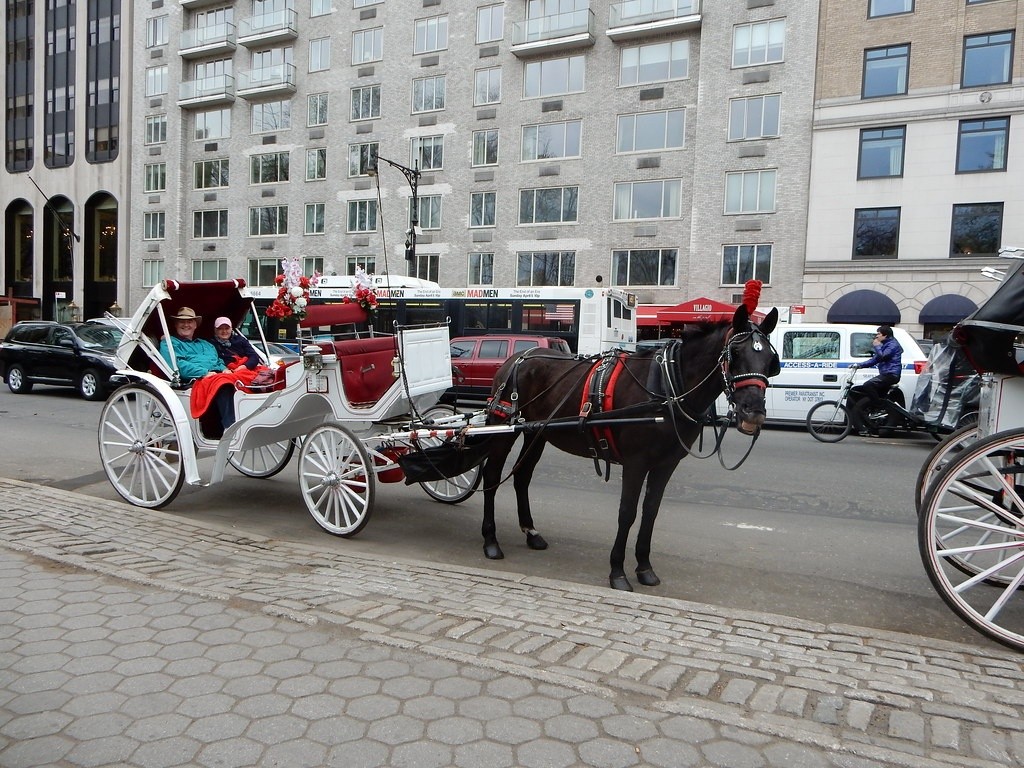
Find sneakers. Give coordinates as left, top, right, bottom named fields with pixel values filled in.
left=859, top=428, right=879, bottom=438
left=870, top=410, right=888, bottom=420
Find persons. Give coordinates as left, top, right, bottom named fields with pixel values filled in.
left=207, top=316, right=274, bottom=394
left=848, top=325, right=904, bottom=437
left=160, top=307, right=235, bottom=440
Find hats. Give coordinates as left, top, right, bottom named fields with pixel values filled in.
left=215, top=317, right=232, bottom=328
left=171, top=307, right=202, bottom=322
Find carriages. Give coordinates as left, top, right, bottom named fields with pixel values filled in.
left=97, top=278, right=782, bottom=594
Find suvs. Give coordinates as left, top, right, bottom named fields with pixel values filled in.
left=0, top=320, right=137, bottom=402
left=792, top=338, right=983, bottom=412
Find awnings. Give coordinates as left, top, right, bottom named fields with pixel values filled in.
left=919, top=294, right=978, bottom=322
left=637, top=306, right=675, bottom=325
left=826, top=289, right=901, bottom=324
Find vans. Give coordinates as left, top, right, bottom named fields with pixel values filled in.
left=435, top=333, right=572, bottom=405
left=703, top=323, right=938, bottom=439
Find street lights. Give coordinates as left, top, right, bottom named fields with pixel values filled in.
left=366, top=147, right=422, bottom=280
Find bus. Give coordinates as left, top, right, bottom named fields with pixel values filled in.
left=239, top=274, right=639, bottom=366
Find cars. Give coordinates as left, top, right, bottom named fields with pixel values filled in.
left=82, top=318, right=134, bottom=345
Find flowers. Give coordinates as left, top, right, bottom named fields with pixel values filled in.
left=342, top=265, right=380, bottom=317
left=264, top=256, right=323, bottom=325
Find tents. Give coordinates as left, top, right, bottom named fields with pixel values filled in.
left=657, top=297, right=766, bottom=340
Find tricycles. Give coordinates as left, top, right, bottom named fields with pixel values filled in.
left=807, top=329, right=982, bottom=452
left=915, top=248, right=1024, bottom=649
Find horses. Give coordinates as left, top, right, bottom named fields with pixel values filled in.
left=482, top=304, right=781, bottom=594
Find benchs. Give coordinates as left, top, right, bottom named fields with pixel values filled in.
left=145, top=363, right=219, bottom=442
left=231, top=359, right=303, bottom=423
left=306, top=337, right=395, bottom=403
